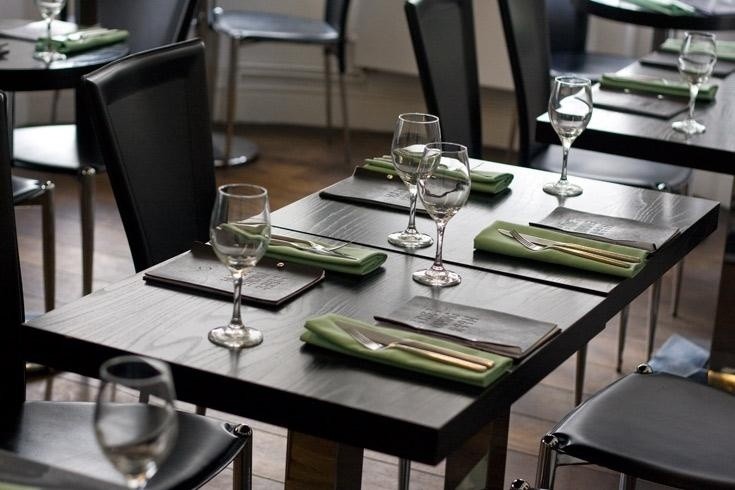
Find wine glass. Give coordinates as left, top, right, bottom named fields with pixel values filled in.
left=413, top=142, right=472, bottom=287
left=31, top=0, right=67, bottom=62
left=208, top=183, right=271, bottom=350
left=388, top=112, right=443, bottom=249
left=672, top=32, right=718, bottom=135
left=92, top=355, right=179, bottom=490
left=543, top=76, right=593, bottom=197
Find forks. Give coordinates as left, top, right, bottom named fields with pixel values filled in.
left=509, top=228, right=631, bottom=268
left=269, top=233, right=348, bottom=251
left=343, top=325, right=488, bottom=372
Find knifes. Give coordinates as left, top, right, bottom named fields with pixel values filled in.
left=497, top=228, right=640, bottom=263
left=270, top=238, right=356, bottom=259
left=334, top=321, right=495, bottom=368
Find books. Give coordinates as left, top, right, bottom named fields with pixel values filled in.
left=639, top=48, right=734, bottom=79
left=317, top=168, right=469, bottom=218
left=373, top=295, right=560, bottom=362
left=586, top=84, right=691, bottom=120
left=529, top=203, right=680, bottom=252
left=0, top=16, right=82, bottom=45
left=146, top=236, right=327, bottom=312
